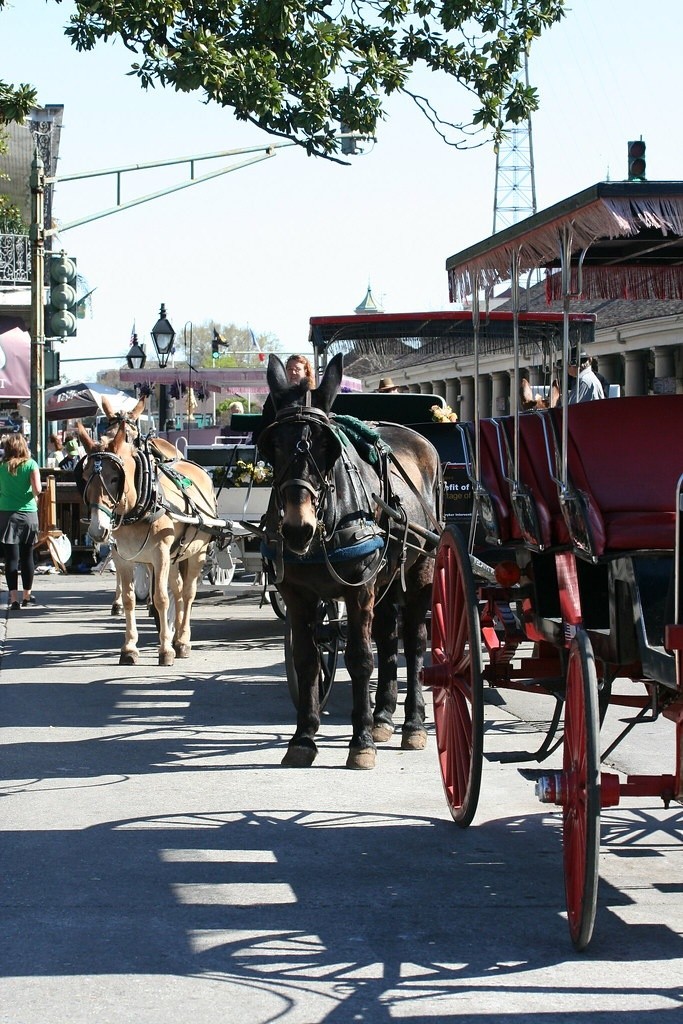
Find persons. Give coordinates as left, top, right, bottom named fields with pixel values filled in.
left=0, top=345, right=608, bottom=612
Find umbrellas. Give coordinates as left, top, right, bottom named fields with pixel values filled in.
left=20, top=380, right=138, bottom=445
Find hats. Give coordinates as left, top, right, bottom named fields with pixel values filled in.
left=558, top=346, right=591, bottom=361
left=67, top=441, right=79, bottom=456
left=374, top=378, right=399, bottom=391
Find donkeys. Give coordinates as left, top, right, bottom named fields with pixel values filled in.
left=101, top=395, right=184, bottom=617
left=74, top=420, right=216, bottom=667
left=267, top=352, right=445, bottom=770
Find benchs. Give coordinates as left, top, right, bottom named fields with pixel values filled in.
left=456, top=392, right=683, bottom=569
left=175, top=436, right=266, bottom=467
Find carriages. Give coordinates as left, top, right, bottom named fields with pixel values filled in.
left=240, top=350, right=503, bottom=771
left=77, top=363, right=365, bottom=666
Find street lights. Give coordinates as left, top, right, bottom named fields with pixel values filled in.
left=127, top=305, right=176, bottom=431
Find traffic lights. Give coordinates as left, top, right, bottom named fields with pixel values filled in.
left=629, top=139, right=647, bottom=182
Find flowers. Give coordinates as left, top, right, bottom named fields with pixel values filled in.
left=207, top=459, right=274, bottom=489
left=431, top=405, right=459, bottom=423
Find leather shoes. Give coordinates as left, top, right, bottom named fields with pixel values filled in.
left=22, top=595, right=35, bottom=607
left=11, top=601, right=20, bottom=610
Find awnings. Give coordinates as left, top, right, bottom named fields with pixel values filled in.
left=0, top=316, right=31, bottom=399
